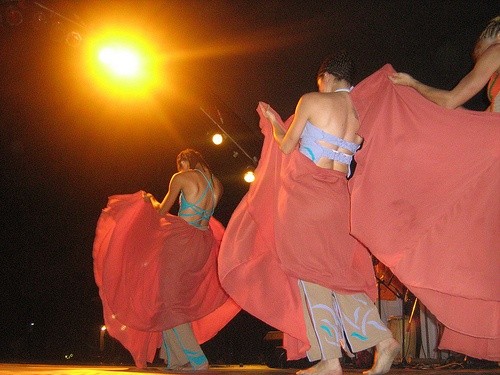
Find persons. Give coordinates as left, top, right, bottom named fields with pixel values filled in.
left=388, top=15, right=500, bottom=367
left=143, top=150, right=222, bottom=369
left=259, top=50, right=401, bottom=375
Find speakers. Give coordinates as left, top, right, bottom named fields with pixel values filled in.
left=262, top=330, right=319, bottom=368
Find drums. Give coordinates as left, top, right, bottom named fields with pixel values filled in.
left=387, top=315, right=416, bottom=365
